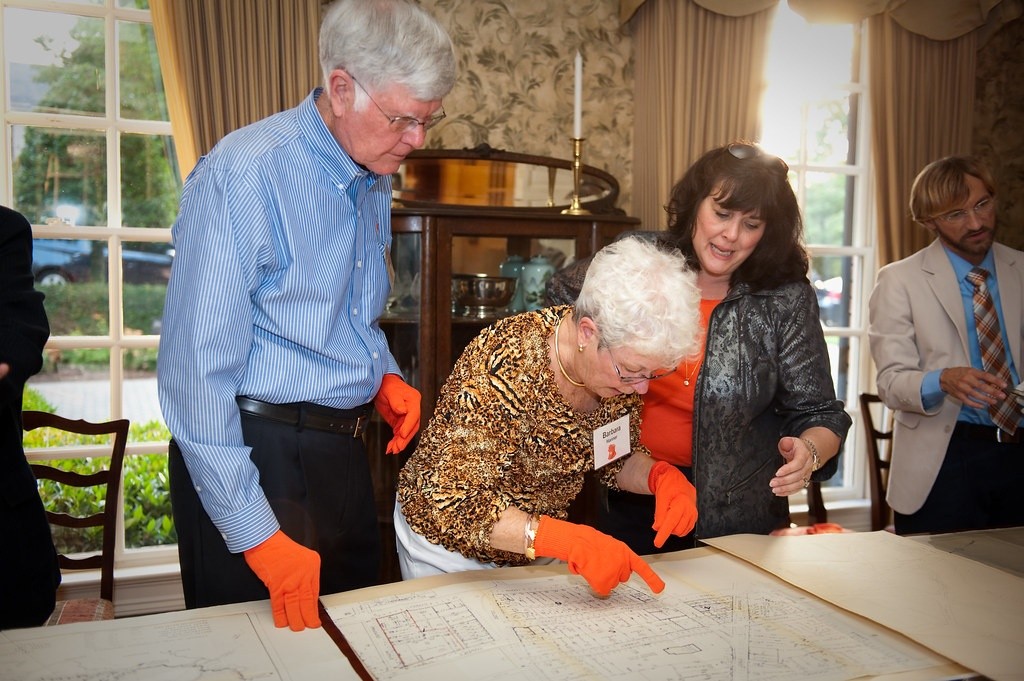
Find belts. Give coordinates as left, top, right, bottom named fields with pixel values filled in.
left=234, top=397, right=368, bottom=438
left=955, top=422, right=1024, bottom=445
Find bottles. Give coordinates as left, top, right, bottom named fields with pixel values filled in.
left=520, top=255, right=557, bottom=312
left=500, top=255, right=528, bottom=313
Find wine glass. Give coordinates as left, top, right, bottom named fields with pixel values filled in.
left=379, top=270, right=421, bottom=319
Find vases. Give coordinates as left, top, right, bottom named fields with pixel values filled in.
left=522, top=254, right=557, bottom=313
left=498, top=255, right=530, bottom=312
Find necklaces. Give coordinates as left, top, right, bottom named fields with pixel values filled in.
left=683, top=351, right=702, bottom=386
left=555, top=318, right=585, bottom=387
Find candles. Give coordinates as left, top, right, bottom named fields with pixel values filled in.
left=573, top=49, right=582, bottom=140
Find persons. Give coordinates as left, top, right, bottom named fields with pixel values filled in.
left=589, top=142, right=854, bottom=556
left=0, top=205, right=64, bottom=632
left=393, top=235, right=701, bottom=605
left=867, top=156, right=1024, bottom=536
left=149, top=0, right=460, bottom=629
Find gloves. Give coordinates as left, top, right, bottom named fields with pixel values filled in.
left=372, top=374, right=423, bottom=454
left=244, top=531, right=324, bottom=630
left=648, top=459, right=699, bottom=549
left=531, top=515, right=670, bottom=598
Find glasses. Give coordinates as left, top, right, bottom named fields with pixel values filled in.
left=602, top=338, right=678, bottom=386
left=717, top=143, right=789, bottom=185
left=932, top=195, right=994, bottom=223
left=340, top=66, right=446, bottom=133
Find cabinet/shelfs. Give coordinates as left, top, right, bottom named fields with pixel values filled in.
left=362, top=205, right=638, bottom=587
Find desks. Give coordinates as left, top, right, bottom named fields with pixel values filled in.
left=0, top=524, right=1024, bottom=681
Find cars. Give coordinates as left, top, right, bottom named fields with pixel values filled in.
left=813, top=276, right=844, bottom=328
left=31, top=240, right=177, bottom=289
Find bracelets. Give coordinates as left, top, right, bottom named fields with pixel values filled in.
left=802, top=437, right=822, bottom=471
left=522, top=512, right=543, bottom=561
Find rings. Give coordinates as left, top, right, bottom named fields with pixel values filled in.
left=803, top=477, right=810, bottom=487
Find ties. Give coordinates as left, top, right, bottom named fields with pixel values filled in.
left=964, top=267, right=1024, bottom=432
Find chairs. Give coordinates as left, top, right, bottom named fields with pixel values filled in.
left=20, top=410, right=131, bottom=624
left=860, top=392, right=897, bottom=533
